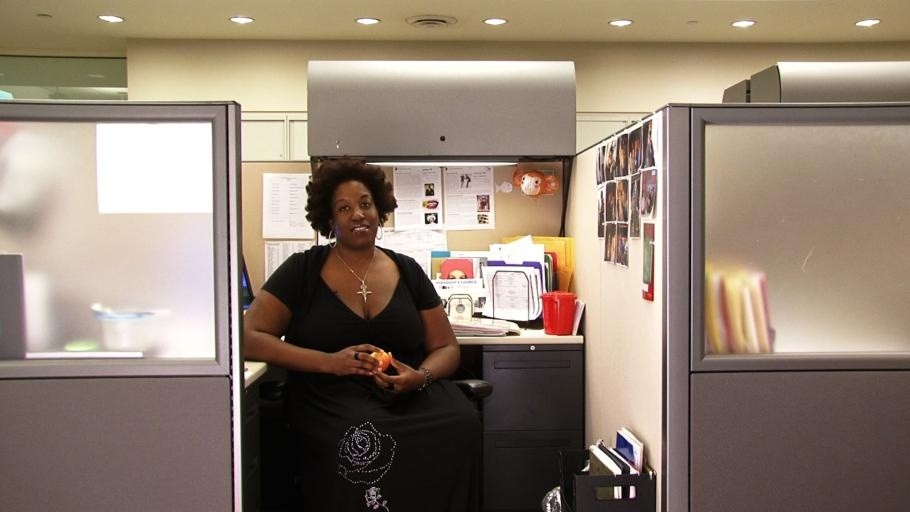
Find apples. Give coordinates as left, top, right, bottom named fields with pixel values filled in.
left=369, top=352, right=390, bottom=372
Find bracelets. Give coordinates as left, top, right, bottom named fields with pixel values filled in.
left=417, top=366, right=433, bottom=392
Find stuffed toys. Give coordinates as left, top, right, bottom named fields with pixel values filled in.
left=511, top=166, right=560, bottom=197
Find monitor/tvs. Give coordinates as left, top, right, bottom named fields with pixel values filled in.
left=242, top=256, right=255, bottom=309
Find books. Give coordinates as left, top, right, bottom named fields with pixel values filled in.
left=588, top=428, right=644, bottom=501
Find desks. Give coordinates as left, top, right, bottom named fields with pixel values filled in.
left=243, top=329, right=587, bottom=512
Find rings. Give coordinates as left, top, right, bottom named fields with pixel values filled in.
left=384, top=383, right=395, bottom=392
left=354, top=351, right=360, bottom=361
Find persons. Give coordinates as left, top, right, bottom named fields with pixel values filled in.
left=241, top=155, right=479, bottom=512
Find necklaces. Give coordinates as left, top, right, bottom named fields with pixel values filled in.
left=334, top=244, right=376, bottom=303
